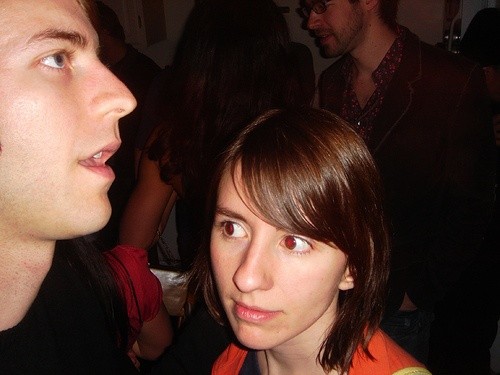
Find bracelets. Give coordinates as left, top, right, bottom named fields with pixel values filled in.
left=157, top=228, right=161, bottom=240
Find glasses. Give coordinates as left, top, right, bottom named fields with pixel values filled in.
left=296, top=2, right=328, bottom=18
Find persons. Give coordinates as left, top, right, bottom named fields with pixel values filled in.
left=0, top=0, right=143, bottom=375
left=95, top=0, right=164, bottom=252
left=456, top=7, right=500, bottom=347
left=176, top=105, right=433, bottom=375
left=57, top=243, right=173, bottom=370
left=300, top=0, right=490, bottom=370
left=118, top=0, right=316, bottom=272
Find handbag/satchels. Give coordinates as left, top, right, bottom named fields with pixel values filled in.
left=150, top=230, right=191, bottom=316
